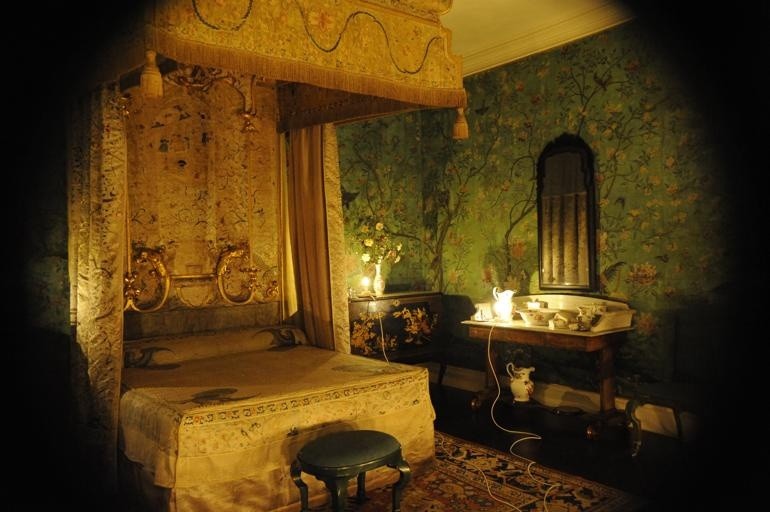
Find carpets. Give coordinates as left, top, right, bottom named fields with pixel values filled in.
left=315, top=430, right=640, bottom=512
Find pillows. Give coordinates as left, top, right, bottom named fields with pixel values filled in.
left=122, top=324, right=308, bottom=368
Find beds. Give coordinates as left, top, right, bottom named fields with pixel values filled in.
left=118, top=300, right=438, bottom=512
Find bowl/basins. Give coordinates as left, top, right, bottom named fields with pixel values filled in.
left=578, top=301, right=607, bottom=321
left=515, top=308, right=560, bottom=326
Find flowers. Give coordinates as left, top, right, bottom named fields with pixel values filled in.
left=350, top=222, right=404, bottom=265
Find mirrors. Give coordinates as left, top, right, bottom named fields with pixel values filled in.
left=535, top=131, right=597, bottom=292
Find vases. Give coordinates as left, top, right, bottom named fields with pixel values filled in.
left=373, top=263, right=386, bottom=297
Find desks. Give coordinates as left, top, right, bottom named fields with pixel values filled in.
left=348, top=291, right=446, bottom=384
left=460, top=294, right=636, bottom=442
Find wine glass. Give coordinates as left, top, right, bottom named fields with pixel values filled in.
left=559, top=309, right=578, bottom=323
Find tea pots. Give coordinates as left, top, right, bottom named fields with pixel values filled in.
left=506, top=362, right=536, bottom=402
left=492, top=287, right=517, bottom=318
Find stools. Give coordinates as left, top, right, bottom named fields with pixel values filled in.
left=289, top=430, right=410, bottom=511
